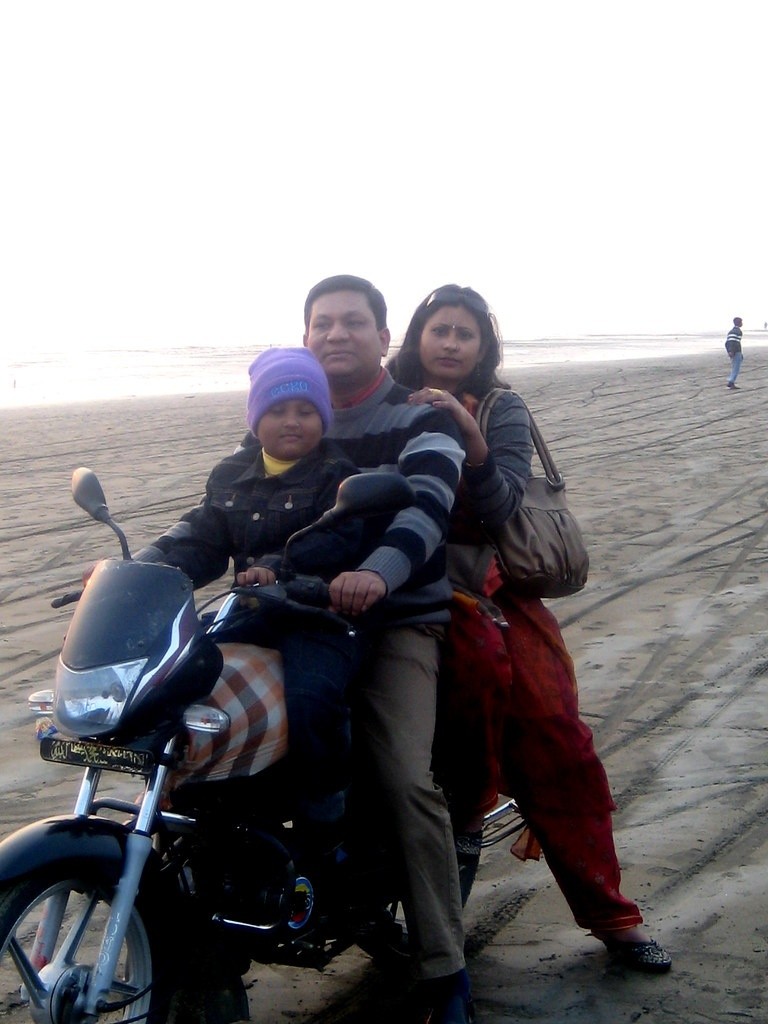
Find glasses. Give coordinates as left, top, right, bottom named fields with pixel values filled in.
left=426, top=291, right=489, bottom=315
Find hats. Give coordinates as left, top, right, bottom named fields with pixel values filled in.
left=248, top=347, right=332, bottom=439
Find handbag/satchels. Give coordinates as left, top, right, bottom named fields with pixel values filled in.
left=477, top=387, right=589, bottom=598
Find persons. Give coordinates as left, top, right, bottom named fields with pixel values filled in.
left=82, top=275, right=672, bottom=1024
left=725, top=317, right=743, bottom=390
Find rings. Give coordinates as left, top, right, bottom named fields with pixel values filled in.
left=428, top=389, right=442, bottom=394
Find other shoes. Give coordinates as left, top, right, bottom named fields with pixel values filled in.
left=727, top=381, right=737, bottom=389
left=433, top=971, right=477, bottom=1024
left=607, top=932, right=672, bottom=971
left=318, top=821, right=347, bottom=868
left=455, top=812, right=483, bottom=868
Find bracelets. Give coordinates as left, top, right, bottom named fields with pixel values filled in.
left=466, top=449, right=491, bottom=467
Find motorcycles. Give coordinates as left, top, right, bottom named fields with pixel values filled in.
left=0, top=468, right=527, bottom=1024
